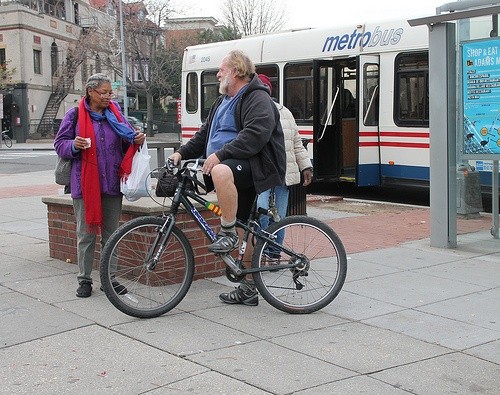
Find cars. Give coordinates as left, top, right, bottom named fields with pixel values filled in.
left=127, top=117, right=158, bottom=136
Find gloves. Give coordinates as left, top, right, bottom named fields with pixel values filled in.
left=303, top=168, right=313, bottom=186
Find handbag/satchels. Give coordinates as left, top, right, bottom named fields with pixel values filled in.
left=155, top=169, right=207, bottom=197
left=120, top=134, right=154, bottom=202
left=55, top=158, right=73, bottom=186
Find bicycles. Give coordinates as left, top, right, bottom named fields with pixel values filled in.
left=2, top=130, right=13, bottom=148
left=98, top=157, right=348, bottom=319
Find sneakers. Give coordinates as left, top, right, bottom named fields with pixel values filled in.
left=219, top=285, right=259, bottom=305
left=206, top=232, right=239, bottom=253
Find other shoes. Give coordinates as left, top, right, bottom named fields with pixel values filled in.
left=76, top=280, right=92, bottom=297
left=260, top=254, right=266, bottom=266
left=268, top=257, right=280, bottom=272
left=100, top=281, right=127, bottom=295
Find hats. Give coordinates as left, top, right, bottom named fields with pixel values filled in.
left=256, top=74, right=272, bottom=89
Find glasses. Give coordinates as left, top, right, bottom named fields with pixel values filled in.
left=90, top=87, right=116, bottom=98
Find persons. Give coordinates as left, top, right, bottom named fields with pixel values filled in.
left=54, top=74, right=145, bottom=298
left=164, top=50, right=286, bottom=306
left=254, top=73, right=313, bottom=272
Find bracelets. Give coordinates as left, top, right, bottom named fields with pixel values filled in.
left=308, top=168, right=313, bottom=172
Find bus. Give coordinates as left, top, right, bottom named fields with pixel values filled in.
left=179, top=16, right=500, bottom=201
